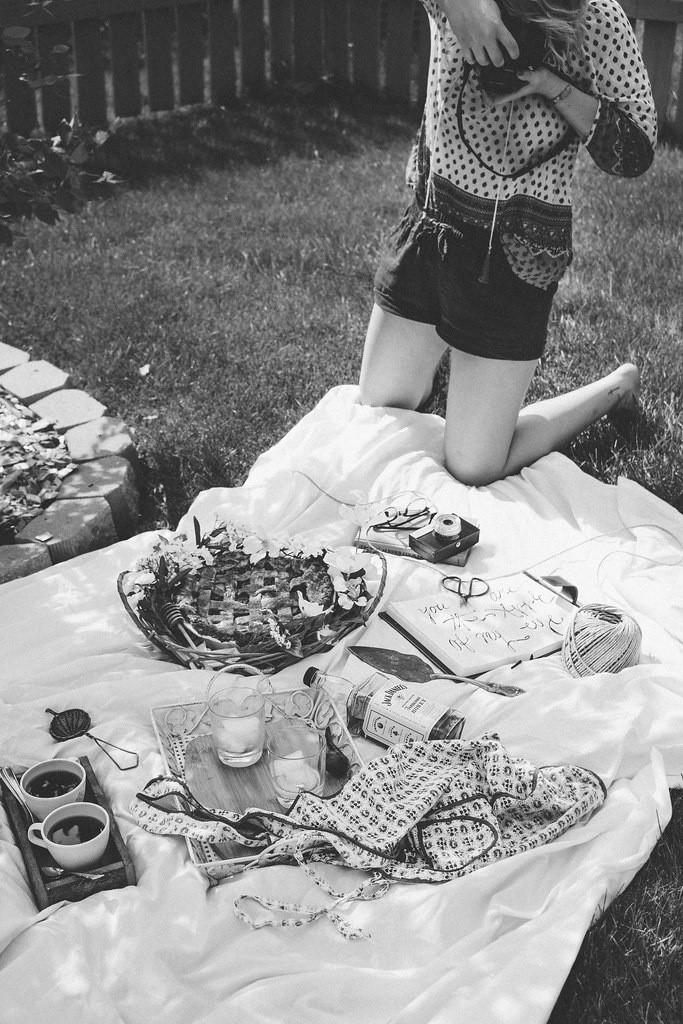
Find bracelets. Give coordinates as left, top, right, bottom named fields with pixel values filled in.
left=543, top=82, right=574, bottom=108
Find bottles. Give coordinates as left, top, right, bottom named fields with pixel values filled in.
left=302, top=666, right=465, bottom=749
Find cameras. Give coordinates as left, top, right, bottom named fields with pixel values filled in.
left=477, top=11, right=543, bottom=91
left=409, top=512, right=480, bottom=560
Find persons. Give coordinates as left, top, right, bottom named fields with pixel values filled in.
left=360, top=1, right=657, bottom=484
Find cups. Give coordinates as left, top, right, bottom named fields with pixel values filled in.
left=209, top=685, right=267, bottom=768
left=268, top=727, right=327, bottom=809
left=27, top=802, right=109, bottom=870
left=17, top=757, right=88, bottom=823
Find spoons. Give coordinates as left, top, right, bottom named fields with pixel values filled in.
left=40, top=860, right=105, bottom=881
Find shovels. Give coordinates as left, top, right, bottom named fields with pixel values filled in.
left=347, top=645, right=525, bottom=698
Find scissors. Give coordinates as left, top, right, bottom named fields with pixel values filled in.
left=441, top=574, right=490, bottom=609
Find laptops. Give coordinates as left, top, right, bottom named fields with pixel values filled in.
left=354, top=503, right=477, bottom=567
